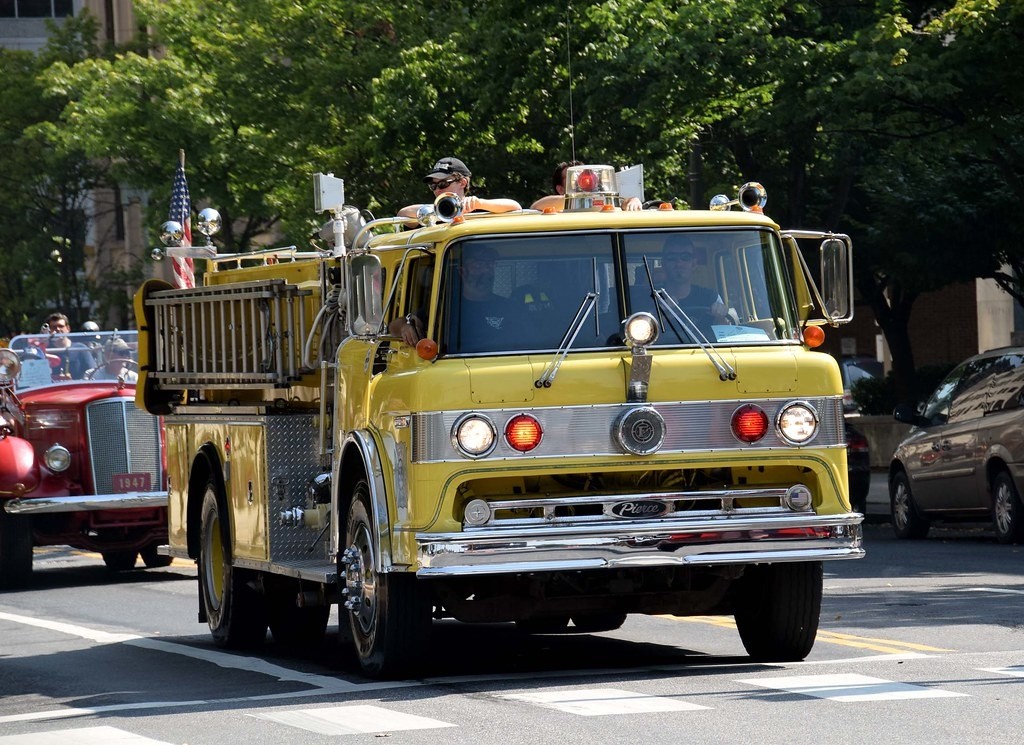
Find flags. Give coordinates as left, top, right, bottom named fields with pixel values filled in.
left=172, top=159, right=197, bottom=292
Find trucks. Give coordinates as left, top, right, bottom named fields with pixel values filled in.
left=0, top=331, right=174, bottom=590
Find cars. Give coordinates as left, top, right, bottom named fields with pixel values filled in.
left=886, top=345, right=1024, bottom=546
left=837, top=388, right=871, bottom=527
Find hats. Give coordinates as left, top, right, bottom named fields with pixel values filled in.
left=103, top=337, right=133, bottom=350
left=422, top=156, right=472, bottom=184
left=80, top=321, right=100, bottom=333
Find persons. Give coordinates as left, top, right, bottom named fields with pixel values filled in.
left=531, top=159, right=643, bottom=315
left=80, top=320, right=105, bottom=367
left=397, top=157, right=522, bottom=231
left=636, top=233, right=730, bottom=345
left=635, top=263, right=652, bottom=285
left=45, top=312, right=100, bottom=381
left=389, top=242, right=531, bottom=354
left=84, top=338, right=139, bottom=383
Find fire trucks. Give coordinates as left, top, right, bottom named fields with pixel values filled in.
left=133, top=163, right=856, bottom=682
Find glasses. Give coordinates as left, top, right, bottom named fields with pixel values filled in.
left=426, top=179, right=460, bottom=192
left=113, top=348, right=131, bottom=357
left=664, top=251, right=694, bottom=262
